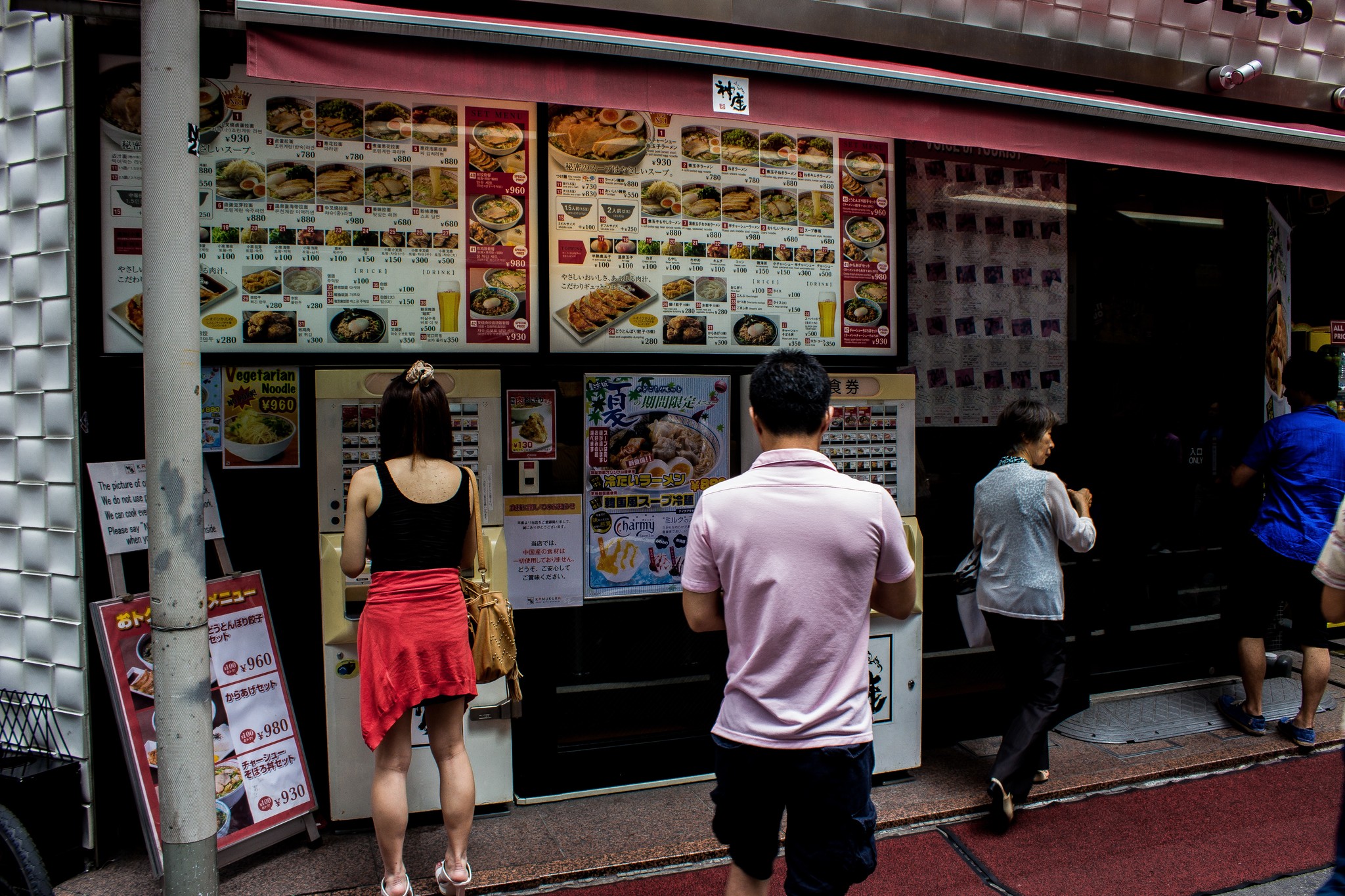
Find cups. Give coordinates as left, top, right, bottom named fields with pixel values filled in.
left=437, top=280, right=461, bottom=332
left=818, top=291, right=836, bottom=337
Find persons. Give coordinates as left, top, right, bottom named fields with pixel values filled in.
left=973, top=400, right=1098, bottom=831
left=681, top=347, right=918, bottom=896
left=341, top=361, right=476, bottom=896
left=1226, top=349, right=1345, bottom=749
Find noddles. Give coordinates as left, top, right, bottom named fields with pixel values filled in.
left=411, top=165, right=458, bottom=206
left=800, top=190, right=833, bottom=226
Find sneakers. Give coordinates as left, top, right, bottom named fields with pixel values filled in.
left=1278, top=717, right=1315, bottom=746
left=1217, top=694, right=1266, bottom=734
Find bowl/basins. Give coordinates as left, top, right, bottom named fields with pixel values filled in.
left=216, top=96, right=523, bottom=230
left=136, top=634, right=153, bottom=670
left=696, top=277, right=727, bottom=302
left=329, top=308, right=386, bottom=343
left=594, top=556, right=682, bottom=582
left=100, top=60, right=233, bottom=151
left=284, top=266, right=323, bottom=295
left=470, top=268, right=526, bottom=319
left=549, top=111, right=655, bottom=174
left=512, top=402, right=544, bottom=423
left=663, top=321, right=706, bottom=344
left=224, top=413, right=296, bottom=461
left=641, top=125, right=884, bottom=248
left=152, top=700, right=216, bottom=731
left=201, top=385, right=209, bottom=405
left=593, top=409, right=724, bottom=493
left=733, top=315, right=778, bottom=346
left=243, top=317, right=295, bottom=343
left=844, top=281, right=887, bottom=326
left=215, top=759, right=245, bottom=839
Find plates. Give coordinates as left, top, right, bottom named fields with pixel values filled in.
left=843, top=237, right=887, bottom=262
left=108, top=263, right=237, bottom=343
left=843, top=171, right=886, bottom=198
left=553, top=272, right=659, bottom=343
left=663, top=276, right=694, bottom=300
left=126, top=667, right=154, bottom=699
left=470, top=143, right=525, bottom=173
left=145, top=724, right=234, bottom=768
left=242, top=266, right=282, bottom=294
left=470, top=219, right=526, bottom=246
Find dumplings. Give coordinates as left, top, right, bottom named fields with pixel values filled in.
left=568, top=283, right=644, bottom=332
left=467, top=146, right=497, bottom=172
left=131, top=669, right=153, bottom=696
left=841, top=173, right=866, bottom=196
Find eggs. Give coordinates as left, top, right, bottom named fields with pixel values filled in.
left=748, top=324, right=765, bottom=336
left=659, top=194, right=698, bottom=214
left=591, top=239, right=636, bottom=254
left=387, top=118, right=404, bottom=131
left=239, top=177, right=259, bottom=191
left=482, top=297, right=501, bottom=308
left=399, top=124, right=412, bottom=138
left=199, top=86, right=220, bottom=106
left=300, top=109, right=315, bottom=120
left=710, top=146, right=720, bottom=155
left=348, top=318, right=369, bottom=332
left=302, top=119, right=315, bottom=128
left=849, top=420, right=868, bottom=424
left=787, top=154, right=797, bottom=165
left=708, top=138, right=721, bottom=146
left=778, top=147, right=791, bottom=158
left=599, top=108, right=644, bottom=133
left=253, top=182, right=266, bottom=197
left=854, top=307, right=868, bottom=316
left=642, top=457, right=693, bottom=489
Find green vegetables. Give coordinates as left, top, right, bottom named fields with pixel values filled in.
left=472, top=125, right=525, bottom=310
left=846, top=155, right=888, bottom=310
left=211, top=98, right=456, bottom=246
left=228, top=415, right=291, bottom=437
left=735, top=323, right=775, bottom=346
left=638, top=128, right=832, bottom=261
left=333, top=312, right=384, bottom=343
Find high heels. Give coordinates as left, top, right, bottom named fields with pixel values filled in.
left=1032, top=771, right=1048, bottom=783
left=434, top=856, right=473, bottom=896
left=987, top=777, right=1014, bottom=825
left=380, top=871, right=414, bottom=896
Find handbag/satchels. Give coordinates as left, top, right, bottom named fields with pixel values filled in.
left=457, top=572, right=524, bottom=702
left=954, top=537, right=996, bottom=648
